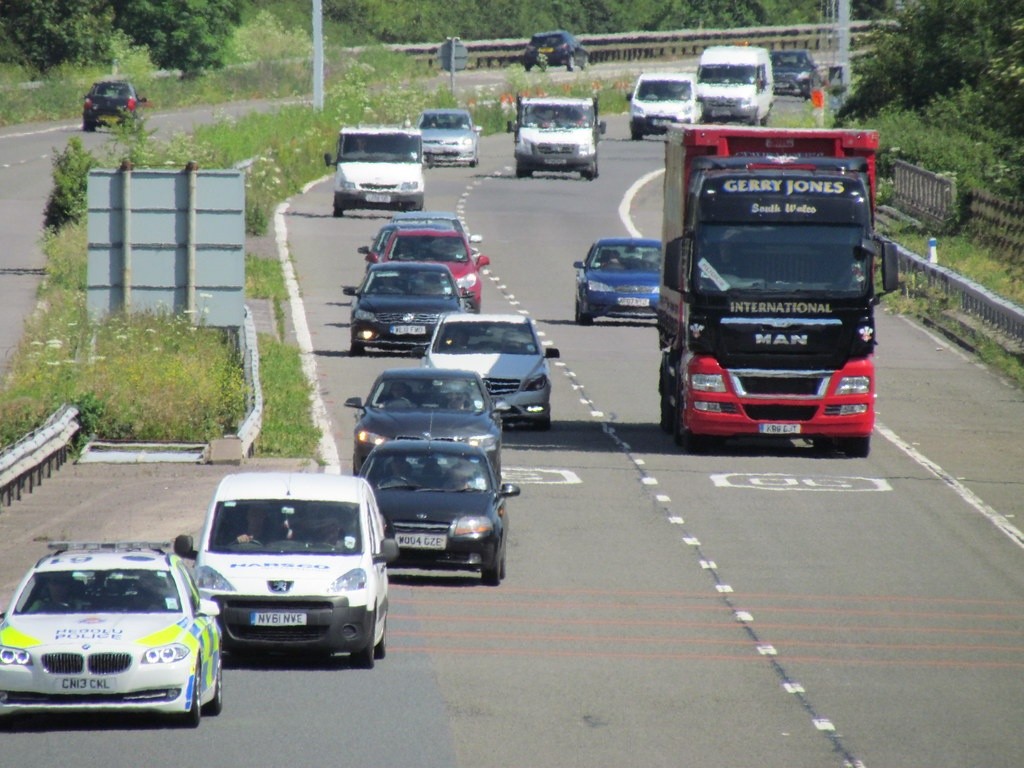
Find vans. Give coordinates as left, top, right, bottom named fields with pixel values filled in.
left=173, top=472, right=400, bottom=670
left=696, top=44, right=776, bottom=127
left=625, top=71, right=703, bottom=141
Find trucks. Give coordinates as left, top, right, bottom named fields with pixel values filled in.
left=507, top=94, right=607, bottom=182
left=324, top=126, right=434, bottom=219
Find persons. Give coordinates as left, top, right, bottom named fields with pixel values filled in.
left=446, top=330, right=469, bottom=351
left=355, top=136, right=369, bottom=153
left=27, top=576, right=82, bottom=612
left=600, top=250, right=620, bottom=269
left=444, top=463, right=472, bottom=489
left=438, top=382, right=470, bottom=410
left=232, top=509, right=269, bottom=546
left=319, top=518, right=341, bottom=549
left=379, top=457, right=419, bottom=487
left=378, top=383, right=417, bottom=408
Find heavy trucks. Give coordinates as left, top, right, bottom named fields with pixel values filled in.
left=653, top=123, right=902, bottom=458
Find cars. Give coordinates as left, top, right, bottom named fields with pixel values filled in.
left=0, top=540, right=222, bottom=730
left=522, top=29, right=590, bottom=72
left=341, top=261, right=476, bottom=357
left=571, top=239, right=664, bottom=326
left=412, top=313, right=561, bottom=430
left=343, top=367, right=505, bottom=489
left=768, top=49, right=823, bottom=103
left=363, top=211, right=489, bottom=315
left=358, top=441, right=522, bottom=589
left=413, top=109, right=483, bottom=167
left=82, top=81, right=147, bottom=132
